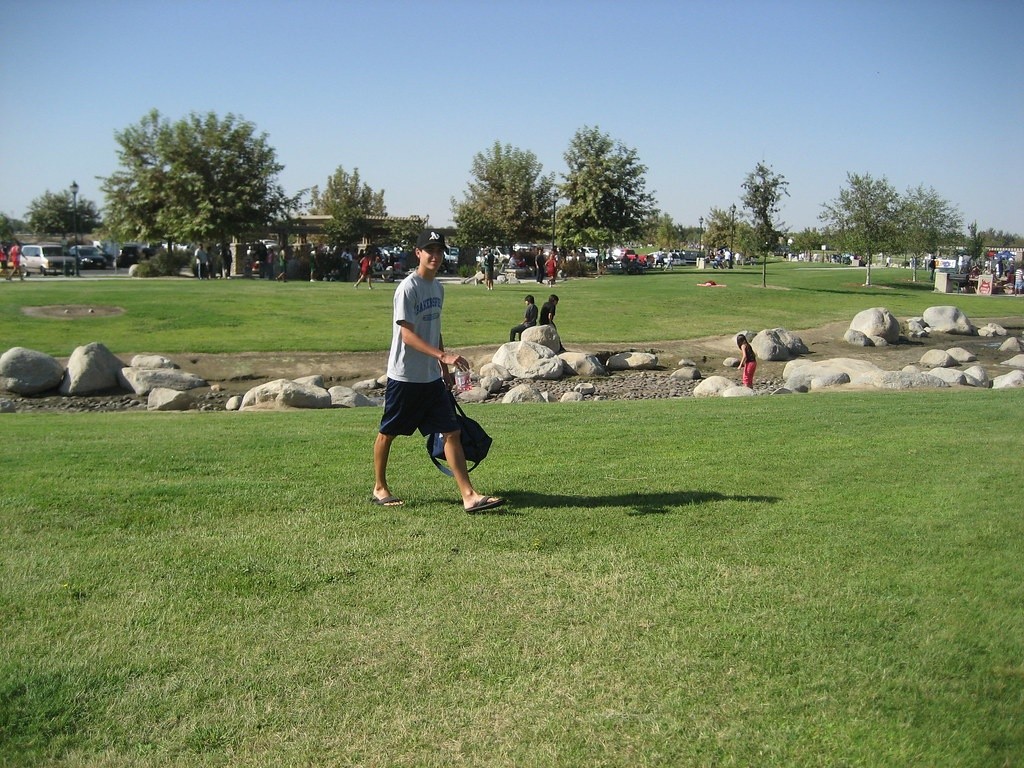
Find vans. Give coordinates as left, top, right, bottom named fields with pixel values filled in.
left=18, top=245, right=76, bottom=276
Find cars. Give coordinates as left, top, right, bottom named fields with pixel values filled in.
left=378, top=244, right=638, bottom=271
left=66, top=243, right=107, bottom=271
left=115, top=245, right=142, bottom=268
left=645, top=249, right=703, bottom=267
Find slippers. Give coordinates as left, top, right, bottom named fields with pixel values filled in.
left=372, top=495, right=405, bottom=508
left=464, top=495, right=507, bottom=512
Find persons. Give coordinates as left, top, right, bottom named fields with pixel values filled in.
left=539, top=294, right=566, bottom=352
left=737, top=334, right=757, bottom=387
left=654, top=248, right=673, bottom=271
left=887, top=256, right=891, bottom=267
left=910, top=256, right=914, bottom=269
left=371, top=232, right=504, bottom=511
left=986, top=259, right=1015, bottom=277
left=735, top=252, right=741, bottom=265
left=0, top=241, right=28, bottom=280
left=195, top=242, right=233, bottom=279
left=715, top=249, right=731, bottom=269
left=310, top=246, right=318, bottom=282
left=622, top=253, right=639, bottom=263
left=535, top=249, right=558, bottom=284
left=1015, top=266, right=1024, bottom=296
left=509, top=253, right=526, bottom=269
left=477, top=248, right=494, bottom=291
left=958, top=253, right=970, bottom=274
left=572, top=248, right=586, bottom=261
left=268, top=245, right=289, bottom=282
left=510, top=295, right=538, bottom=342
left=783, top=252, right=792, bottom=262
left=341, top=249, right=382, bottom=291
left=929, top=256, right=936, bottom=280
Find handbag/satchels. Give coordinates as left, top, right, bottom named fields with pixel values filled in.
left=426, top=389, right=493, bottom=476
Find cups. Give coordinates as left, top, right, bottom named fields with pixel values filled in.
left=454, top=355, right=472, bottom=391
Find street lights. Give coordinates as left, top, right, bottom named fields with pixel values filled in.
left=69, top=179, right=82, bottom=279
left=728, top=203, right=736, bottom=269
left=550, top=190, right=559, bottom=252
left=697, top=217, right=704, bottom=264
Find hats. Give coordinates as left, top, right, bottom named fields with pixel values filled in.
left=415, top=229, right=447, bottom=252
